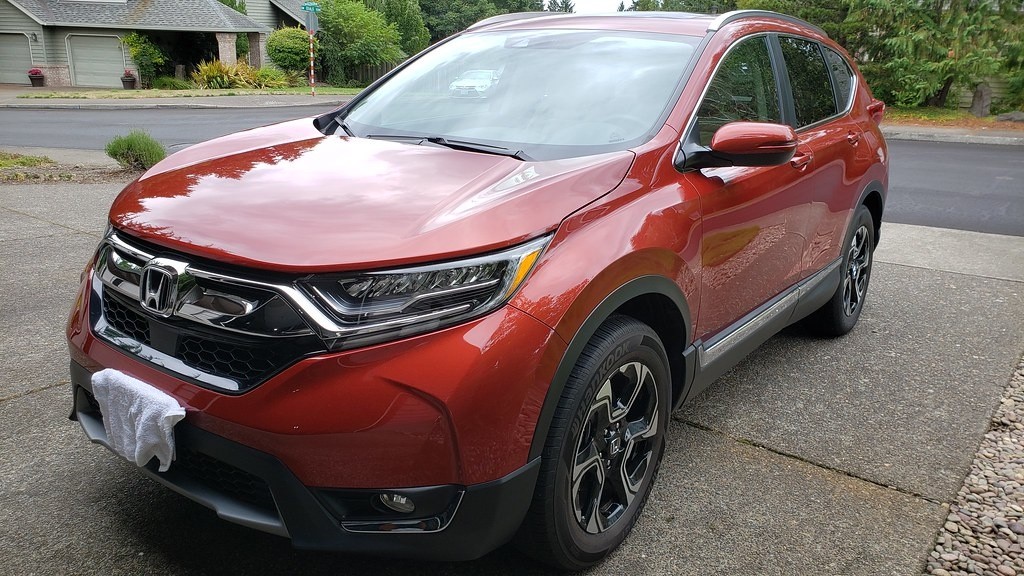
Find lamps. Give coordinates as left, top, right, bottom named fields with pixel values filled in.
left=31, top=32, right=37, bottom=41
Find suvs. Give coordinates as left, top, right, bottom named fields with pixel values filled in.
left=65, top=8, right=888, bottom=576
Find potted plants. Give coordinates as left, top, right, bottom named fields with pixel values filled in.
left=26, top=69, right=44, bottom=87
left=121, top=70, right=136, bottom=90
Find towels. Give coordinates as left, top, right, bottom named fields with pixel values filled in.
left=91, top=368, right=186, bottom=472
